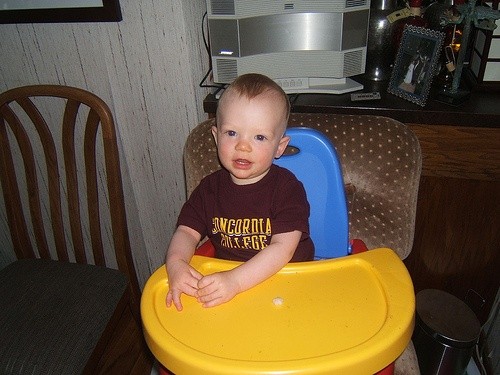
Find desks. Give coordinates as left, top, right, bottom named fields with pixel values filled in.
left=202, top=64, right=499, bottom=325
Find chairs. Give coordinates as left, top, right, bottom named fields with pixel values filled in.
left=0, top=83, right=156, bottom=375
left=271, top=127, right=352, bottom=264
left=182, top=112, right=422, bottom=375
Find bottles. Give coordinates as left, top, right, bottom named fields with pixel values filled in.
left=396, top=0, right=428, bottom=52
left=365, top=0, right=395, bottom=81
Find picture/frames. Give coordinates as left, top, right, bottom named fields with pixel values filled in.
left=387, top=24, right=446, bottom=107
left=0, top=0, right=122, bottom=25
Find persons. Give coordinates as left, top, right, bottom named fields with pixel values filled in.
left=412, top=58, right=421, bottom=85
left=403, top=59, right=415, bottom=84
left=164, top=73, right=317, bottom=315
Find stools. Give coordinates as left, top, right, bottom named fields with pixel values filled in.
left=415, top=288, right=481, bottom=375
